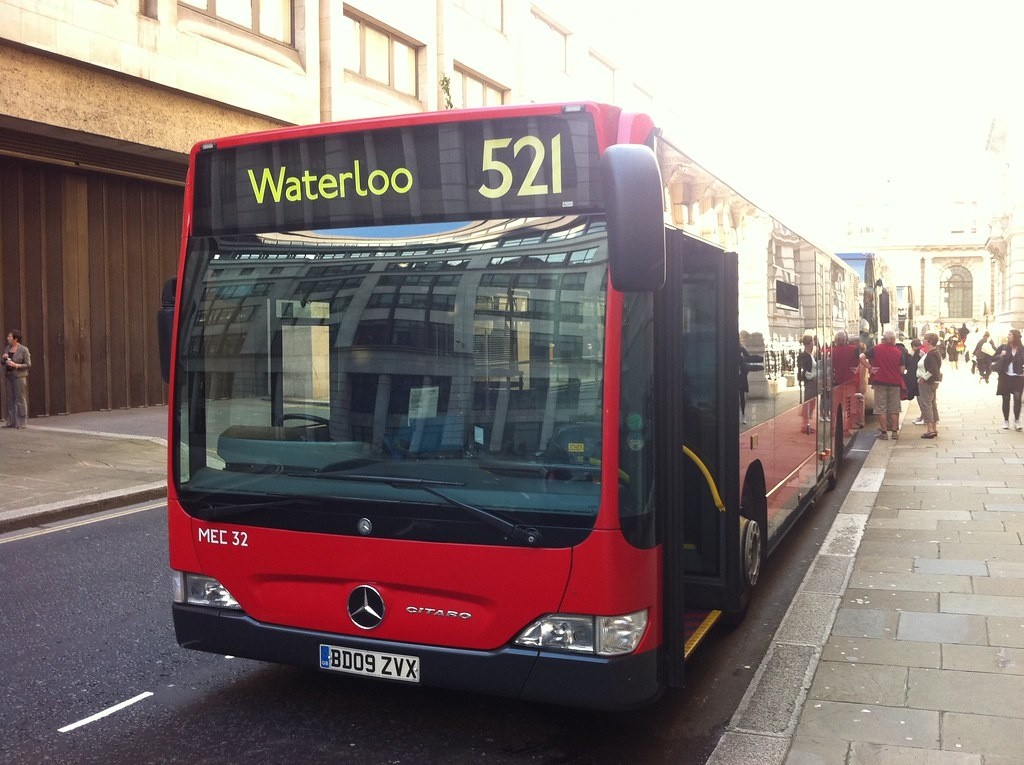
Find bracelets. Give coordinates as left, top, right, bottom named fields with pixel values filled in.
left=16, top=363, right=18, bottom=370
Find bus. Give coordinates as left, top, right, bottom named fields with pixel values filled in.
left=157, top=101, right=874, bottom=710
left=834, top=253, right=898, bottom=344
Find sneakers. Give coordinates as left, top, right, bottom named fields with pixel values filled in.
left=741, top=415, right=747, bottom=425
left=873, top=431, right=889, bottom=441
left=1015, top=421, right=1022, bottom=431
left=891, top=430, right=899, bottom=439
left=1003, top=419, right=1009, bottom=430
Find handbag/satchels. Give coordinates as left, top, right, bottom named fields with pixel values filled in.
left=970, top=359, right=977, bottom=374
left=988, top=344, right=1007, bottom=371
left=981, top=337, right=996, bottom=356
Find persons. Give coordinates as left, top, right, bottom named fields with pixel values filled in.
left=910, top=338, right=930, bottom=426
left=738, top=312, right=878, bottom=463
left=930, top=320, right=1001, bottom=384
left=859, top=329, right=906, bottom=440
left=915, top=333, right=942, bottom=439
left=0, top=328, right=33, bottom=429
left=990, top=329, right=1024, bottom=431
left=876, top=342, right=909, bottom=431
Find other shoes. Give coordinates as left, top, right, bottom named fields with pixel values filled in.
left=820, top=415, right=831, bottom=421
left=912, top=416, right=927, bottom=425
left=921, top=431, right=938, bottom=439
left=801, top=426, right=817, bottom=434
left=19, top=425, right=29, bottom=430
left=1, top=422, right=17, bottom=430
